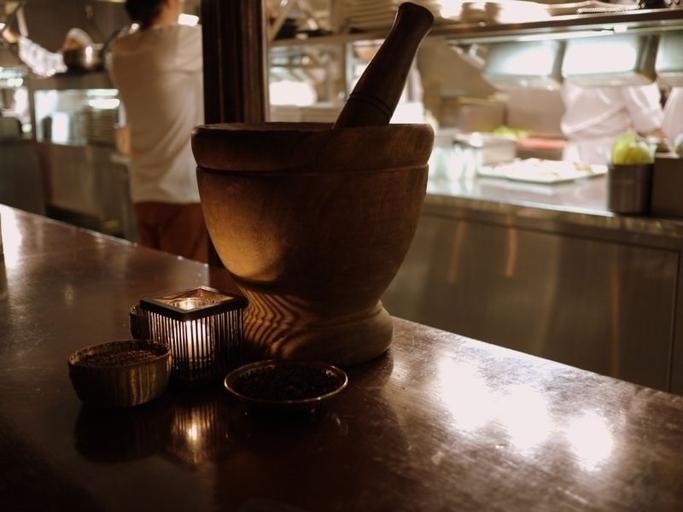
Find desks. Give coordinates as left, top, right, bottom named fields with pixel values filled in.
left=1, top=200, right=682, bottom=512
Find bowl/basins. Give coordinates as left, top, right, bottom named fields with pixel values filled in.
left=224, top=360, right=349, bottom=427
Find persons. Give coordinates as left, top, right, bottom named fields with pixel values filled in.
left=111, top=0, right=210, bottom=265
left=560, top=83, right=683, bottom=164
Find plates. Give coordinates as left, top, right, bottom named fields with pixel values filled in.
left=350, top=2, right=440, bottom=29
left=86, top=106, right=118, bottom=146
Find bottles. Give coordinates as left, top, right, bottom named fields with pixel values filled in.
left=607, top=164, right=652, bottom=213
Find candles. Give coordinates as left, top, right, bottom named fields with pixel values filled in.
left=168, top=297, right=211, bottom=359
